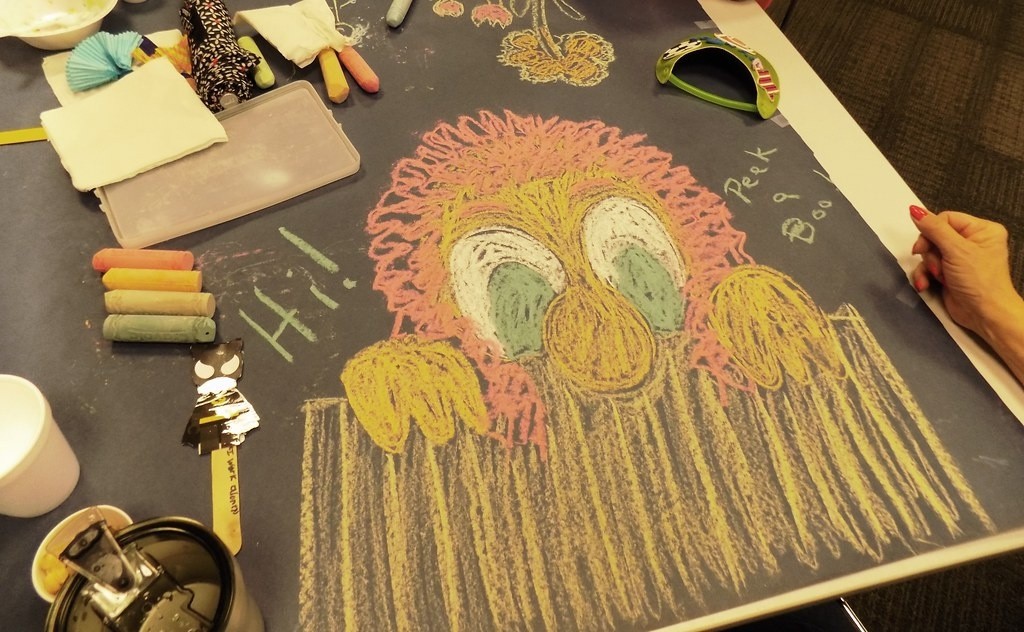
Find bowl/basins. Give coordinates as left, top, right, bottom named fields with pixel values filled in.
left=1, top=0, right=118, bottom=49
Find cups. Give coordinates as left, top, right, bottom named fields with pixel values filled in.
left=0, top=374, right=80, bottom=518
left=31, top=504, right=134, bottom=603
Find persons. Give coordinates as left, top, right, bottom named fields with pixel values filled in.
left=908, top=203, right=1024, bottom=390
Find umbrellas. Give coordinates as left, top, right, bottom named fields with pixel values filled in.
left=178, top=0, right=262, bottom=114
